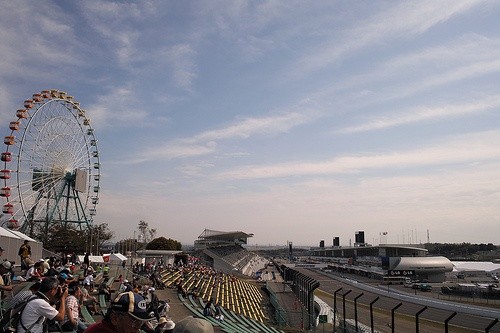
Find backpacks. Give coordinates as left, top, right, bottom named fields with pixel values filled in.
left=0, top=296, right=48, bottom=333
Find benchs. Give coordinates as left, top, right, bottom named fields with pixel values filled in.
left=0, top=270, right=285, bottom=333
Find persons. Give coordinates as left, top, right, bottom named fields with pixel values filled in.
left=0, top=240, right=264, bottom=333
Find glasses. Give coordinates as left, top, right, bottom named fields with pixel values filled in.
left=120, top=314, right=146, bottom=329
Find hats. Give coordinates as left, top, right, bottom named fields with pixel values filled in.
left=172, top=316, right=214, bottom=333
left=112, top=291, right=156, bottom=321
left=2, top=261, right=11, bottom=269
left=149, top=287, right=155, bottom=291
left=156, top=317, right=167, bottom=324
left=163, top=320, right=175, bottom=330
left=122, top=280, right=129, bottom=283
left=39, top=258, right=45, bottom=262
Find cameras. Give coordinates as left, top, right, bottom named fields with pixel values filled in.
left=54, top=287, right=75, bottom=298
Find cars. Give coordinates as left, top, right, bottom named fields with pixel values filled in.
left=403, top=279, right=433, bottom=292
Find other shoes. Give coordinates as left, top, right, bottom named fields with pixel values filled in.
left=94, top=312, right=102, bottom=315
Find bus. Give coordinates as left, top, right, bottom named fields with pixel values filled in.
left=380, top=276, right=412, bottom=284
